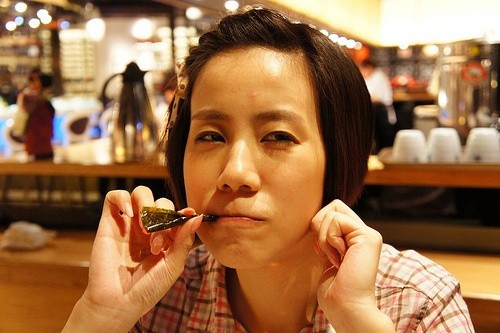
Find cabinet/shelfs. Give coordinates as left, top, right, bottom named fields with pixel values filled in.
left=0, top=162, right=500, bottom=333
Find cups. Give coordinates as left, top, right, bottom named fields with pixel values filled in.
left=462, top=126, right=500, bottom=166
left=392, top=131, right=425, bottom=165
left=425, top=128, right=460, bottom=165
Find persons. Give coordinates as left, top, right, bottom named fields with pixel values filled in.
left=0, top=67, right=56, bottom=162
left=112, top=62, right=160, bottom=163
left=361, top=59, right=397, bottom=147
left=60, top=8, right=475, bottom=333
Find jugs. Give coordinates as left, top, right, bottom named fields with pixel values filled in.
left=99, top=63, right=158, bottom=167
